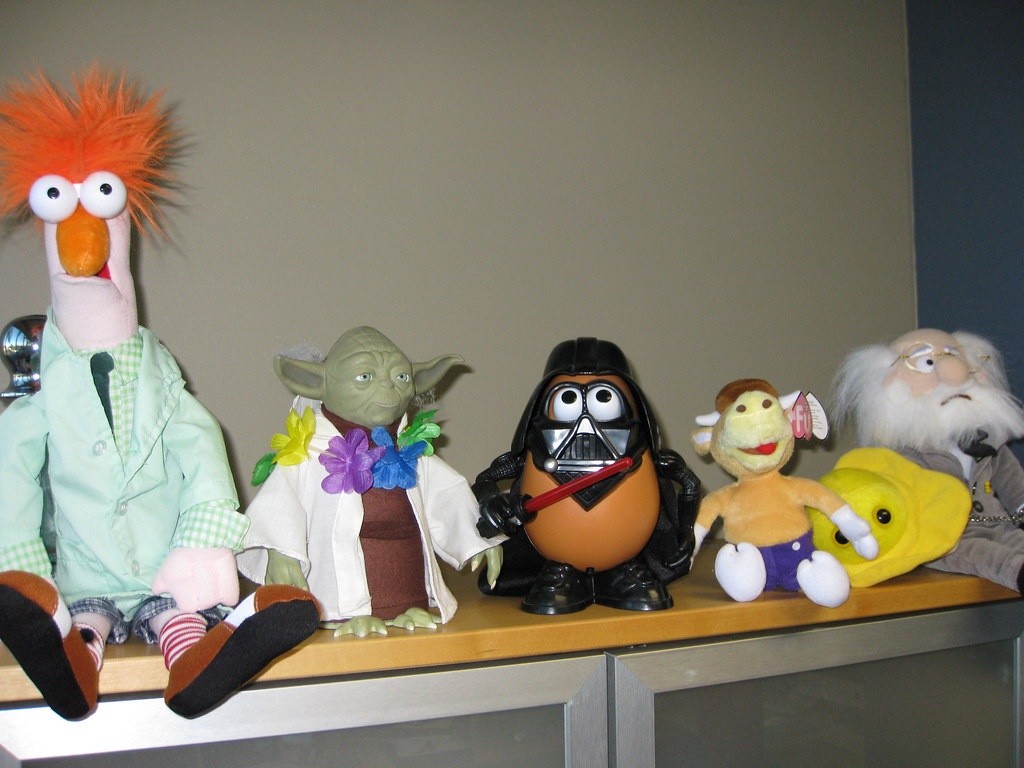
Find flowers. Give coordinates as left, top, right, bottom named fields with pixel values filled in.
left=250, top=408, right=442, bottom=494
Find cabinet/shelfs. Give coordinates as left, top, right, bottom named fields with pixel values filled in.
left=0, top=568, right=1024, bottom=768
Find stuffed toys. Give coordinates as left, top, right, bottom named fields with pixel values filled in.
left=689, top=328, right=1024, bottom=607
left=1, top=71, right=323, bottom=722
